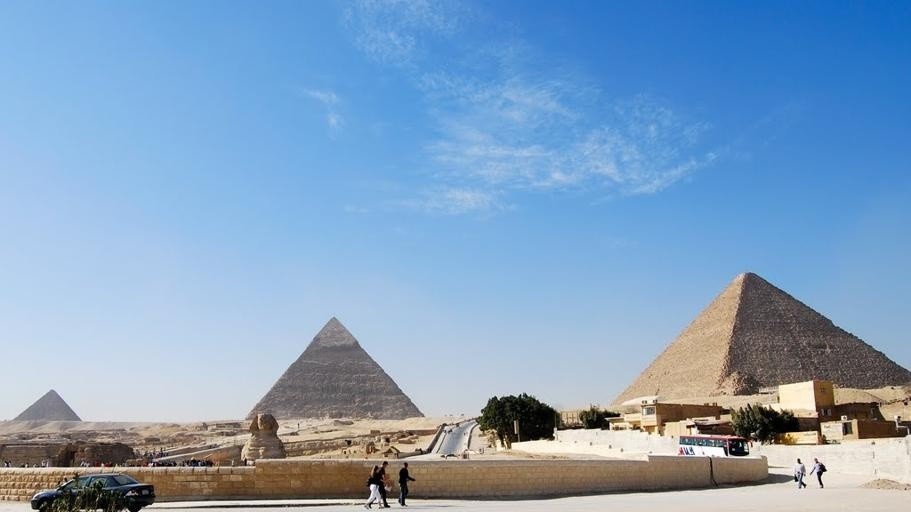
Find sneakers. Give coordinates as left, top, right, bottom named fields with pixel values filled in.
left=799, top=484, right=806, bottom=489
left=821, top=484, right=823, bottom=488
left=364, top=500, right=407, bottom=509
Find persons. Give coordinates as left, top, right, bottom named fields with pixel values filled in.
left=364, top=464, right=385, bottom=509
left=398, top=462, right=417, bottom=507
left=810, top=456, right=828, bottom=488
left=369, top=461, right=392, bottom=508
left=4, top=446, right=217, bottom=467
left=794, top=458, right=808, bottom=489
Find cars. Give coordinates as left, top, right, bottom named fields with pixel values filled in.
left=30, top=470, right=156, bottom=512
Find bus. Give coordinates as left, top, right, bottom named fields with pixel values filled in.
left=676, top=434, right=750, bottom=458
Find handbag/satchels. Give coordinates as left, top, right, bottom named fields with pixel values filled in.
left=366, top=477, right=372, bottom=486
left=819, top=465, right=827, bottom=472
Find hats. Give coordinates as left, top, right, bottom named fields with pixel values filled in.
left=383, top=461, right=389, bottom=465
